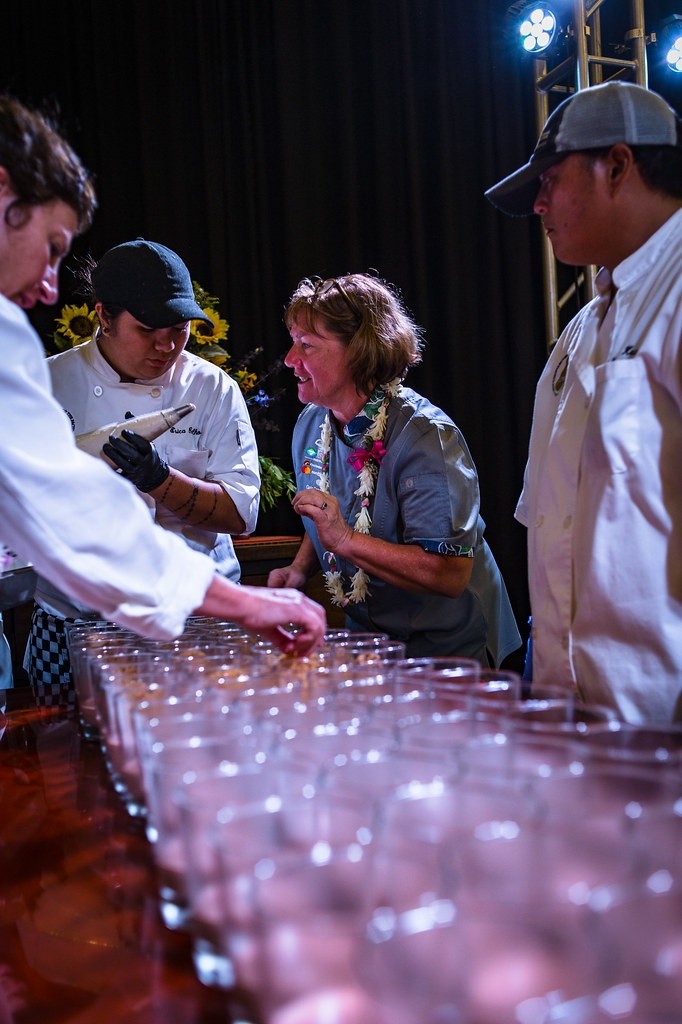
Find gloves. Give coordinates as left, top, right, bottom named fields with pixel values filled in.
left=102, top=428, right=169, bottom=493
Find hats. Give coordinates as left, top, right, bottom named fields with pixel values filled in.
left=484, top=80, right=678, bottom=217
left=91, top=236, right=215, bottom=329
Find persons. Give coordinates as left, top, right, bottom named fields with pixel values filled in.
left=22, top=241, right=262, bottom=707
left=267, top=274, right=521, bottom=671
left=1, top=99, right=327, bottom=743
left=485, top=81, right=682, bottom=723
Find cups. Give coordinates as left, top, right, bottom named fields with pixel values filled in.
left=62, top=612, right=682, bottom=1024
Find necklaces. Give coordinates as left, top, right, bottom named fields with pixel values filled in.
left=316, top=382, right=396, bottom=606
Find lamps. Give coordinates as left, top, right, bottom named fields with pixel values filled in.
left=502, top=0, right=563, bottom=63
left=656, top=14, right=682, bottom=73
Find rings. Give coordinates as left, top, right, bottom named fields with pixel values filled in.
left=321, top=503, right=327, bottom=510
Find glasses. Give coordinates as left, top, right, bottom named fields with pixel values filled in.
left=317, top=277, right=364, bottom=322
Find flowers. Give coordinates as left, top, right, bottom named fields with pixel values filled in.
left=54, top=282, right=298, bottom=510
